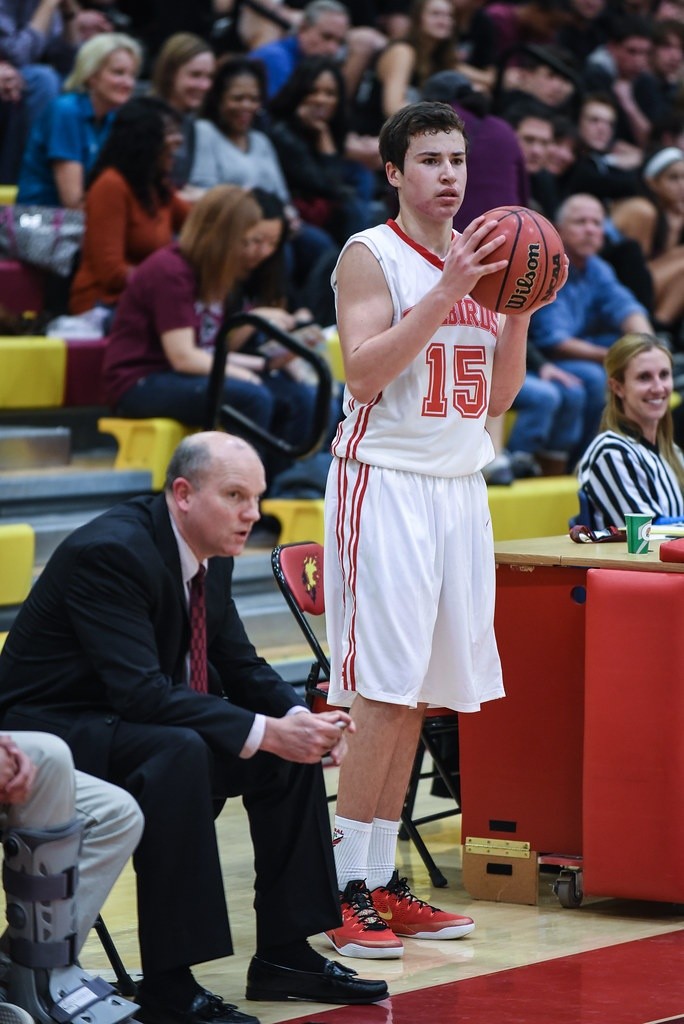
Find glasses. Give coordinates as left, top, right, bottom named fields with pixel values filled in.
left=320, top=31, right=346, bottom=47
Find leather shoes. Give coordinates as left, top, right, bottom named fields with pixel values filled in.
left=244, top=955, right=390, bottom=1004
left=132, top=986, right=262, bottom=1024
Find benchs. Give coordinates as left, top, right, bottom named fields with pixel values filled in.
left=0, top=190, right=580, bottom=606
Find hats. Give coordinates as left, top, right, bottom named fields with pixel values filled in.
left=420, top=70, right=472, bottom=101
left=527, top=41, right=579, bottom=82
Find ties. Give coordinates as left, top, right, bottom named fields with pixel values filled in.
left=190, top=564, right=208, bottom=694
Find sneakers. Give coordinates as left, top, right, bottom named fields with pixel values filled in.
left=323, top=878, right=404, bottom=959
left=371, top=869, right=475, bottom=940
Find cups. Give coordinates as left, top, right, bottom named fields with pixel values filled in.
left=624, top=513, right=653, bottom=554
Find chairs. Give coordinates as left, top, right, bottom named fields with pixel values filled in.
left=271, top=540, right=460, bottom=888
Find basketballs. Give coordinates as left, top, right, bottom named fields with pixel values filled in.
left=466, top=205, right=566, bottom=315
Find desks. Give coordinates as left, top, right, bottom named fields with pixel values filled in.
left=459, top=525, right=684, bottom=905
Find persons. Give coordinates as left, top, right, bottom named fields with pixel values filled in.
left=0, top=430, right=391, bottom=1024
left=0, top=0, right=684, bottom=528
left=321, top=101, right=571, bottom=957
